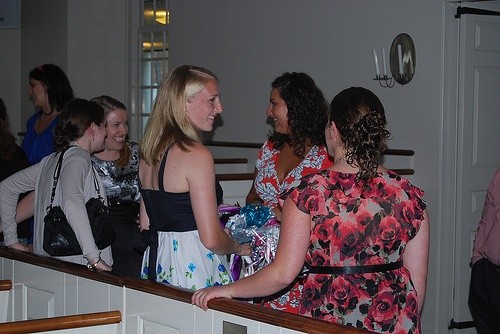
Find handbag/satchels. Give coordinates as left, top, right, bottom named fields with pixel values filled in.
left=43, top=145, right=116, bottom=256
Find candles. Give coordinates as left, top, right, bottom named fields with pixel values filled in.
left=373, top=50, right=379, bottom=75
left=382, top=47, right=387, bottom=75
left=397, top=43, right=404, bottom=74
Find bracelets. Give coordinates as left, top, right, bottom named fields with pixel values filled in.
left=87, top=257, right=102, bottom=272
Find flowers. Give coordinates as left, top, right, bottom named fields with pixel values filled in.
left=225, top=202, right=271, bottom=246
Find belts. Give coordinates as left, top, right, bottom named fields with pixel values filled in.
left=308, top=261, right=404, bottom=274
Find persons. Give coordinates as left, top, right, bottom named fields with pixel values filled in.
left=189, top=86, right=431, bottom=334
left=21, top=63, right=74, bottom=166
left=87, top=94, right=146, bottom=280
left=138, top=65, right=254, bottom=293
left=467, top=166, right=500, bottom=334
left=246, top=72, right=329, bottom=229
left=0, top=98, right=36, bottom=251
left=0, top=98, right=116, bottom=274
